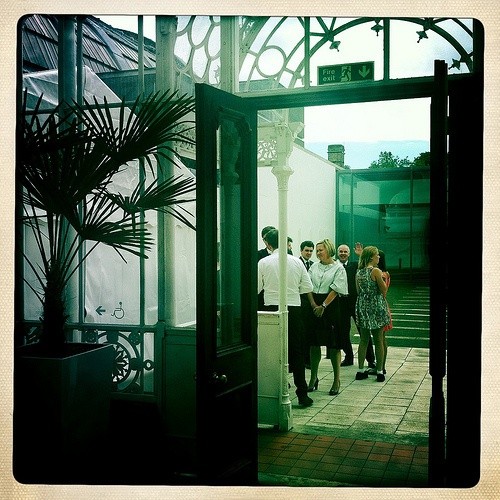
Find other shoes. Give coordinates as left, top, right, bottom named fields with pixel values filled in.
left=305, top=361, right=311, bottom=369
left=369, top=367, right=386, bottom=374
left=366, top=362, right=376, bottom=373
left=356, top=371, right=369, bottom=380
left=340, top=360, right=352, bottom=366
left=299, top=395, right=313, bottom=404
left=376, top=373, right=385, bottom=382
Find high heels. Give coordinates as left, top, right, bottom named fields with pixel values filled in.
left=307, top=378, right=318, bottom=391
left=329, top=380, right=340, bottom=395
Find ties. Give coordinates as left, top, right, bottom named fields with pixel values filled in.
left=343, top=263, right=347, bottom=268
left=306, top=262, right=309, bottom=271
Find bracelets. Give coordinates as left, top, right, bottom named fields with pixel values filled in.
left=321, top=302, right=327, bottom=308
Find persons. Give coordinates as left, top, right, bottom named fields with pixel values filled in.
left=302, top=239, right=348, bottom=396
left=254, top=226, right=314, bottom=405
left=333, top=242, right=392, bottom=382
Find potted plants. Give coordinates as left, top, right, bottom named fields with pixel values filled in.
left=17, top=85, right=201, bottom=467
